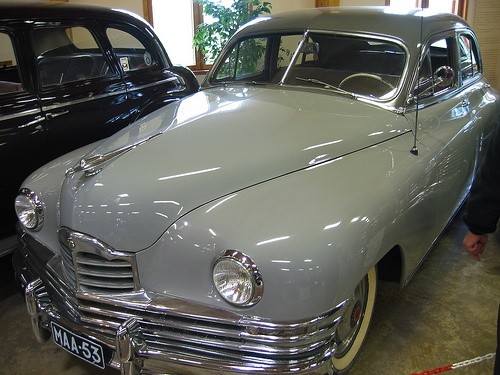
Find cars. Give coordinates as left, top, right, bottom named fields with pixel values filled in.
left=0, top=5, right=199, bottom=299
left=15, top=6, right=500, bottom=375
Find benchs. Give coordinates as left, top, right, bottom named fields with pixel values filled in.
left=328, top=50, right=452, bottom=77
left=37, top=54, right=146, bottom=87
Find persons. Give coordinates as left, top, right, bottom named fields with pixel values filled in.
left=462, top=105, right=500, bottom=374
